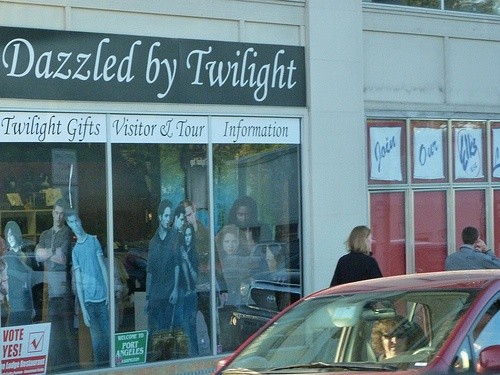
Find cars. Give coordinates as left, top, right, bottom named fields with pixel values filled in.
left=22, top=233, right=78, bottom=305
left=209, top=267, right=500, bottom=375
left=110, top=240, right=150, bottom=293
left=227, top=241, right=303, bottom=349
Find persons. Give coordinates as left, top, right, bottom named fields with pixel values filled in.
left=443, top=224, right=500, bottom=271
left=330, top=225, right=383, bottom=363
left=5, top=195, right=272, bottom=368
left=371, top=316, right=418, bottom=370
left=227, top=196, right=290, bottom=329
left=449, top=295, right=500, bottom=372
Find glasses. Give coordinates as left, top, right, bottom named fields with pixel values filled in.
left=383, top=334, right=408, bottom=339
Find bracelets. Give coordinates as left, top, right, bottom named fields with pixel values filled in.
left=52, top=246, right=56, bottom=255
left=189, top=265, right=192, bottom=270
left=190, top=268, right=194, bottom=273
left=484, top=248, right=492, bottom=254
